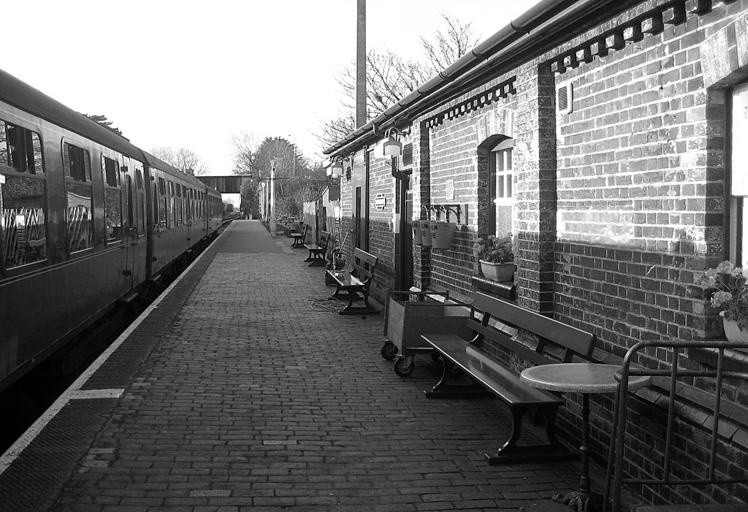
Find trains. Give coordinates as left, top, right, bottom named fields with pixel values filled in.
left=1, top=67, right=226, bottom=396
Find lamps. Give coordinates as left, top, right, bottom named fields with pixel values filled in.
left=374, top=126, right=412, bottom=160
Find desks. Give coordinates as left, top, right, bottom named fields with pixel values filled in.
left=519, top=363, right=653, bottom=512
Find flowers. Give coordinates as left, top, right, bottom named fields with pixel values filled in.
left=473, top=233, right=515, bottom=265
left=696, top=260, right=748, bottom=331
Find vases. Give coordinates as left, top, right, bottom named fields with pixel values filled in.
left=479, top=260, right=515, bottom=282
left=719, top=310, right=748, bottom=345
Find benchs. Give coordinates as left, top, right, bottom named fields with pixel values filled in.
left=288, top=222, right=379, bottom=316
left=421, top=291, right=598, bottom=467
